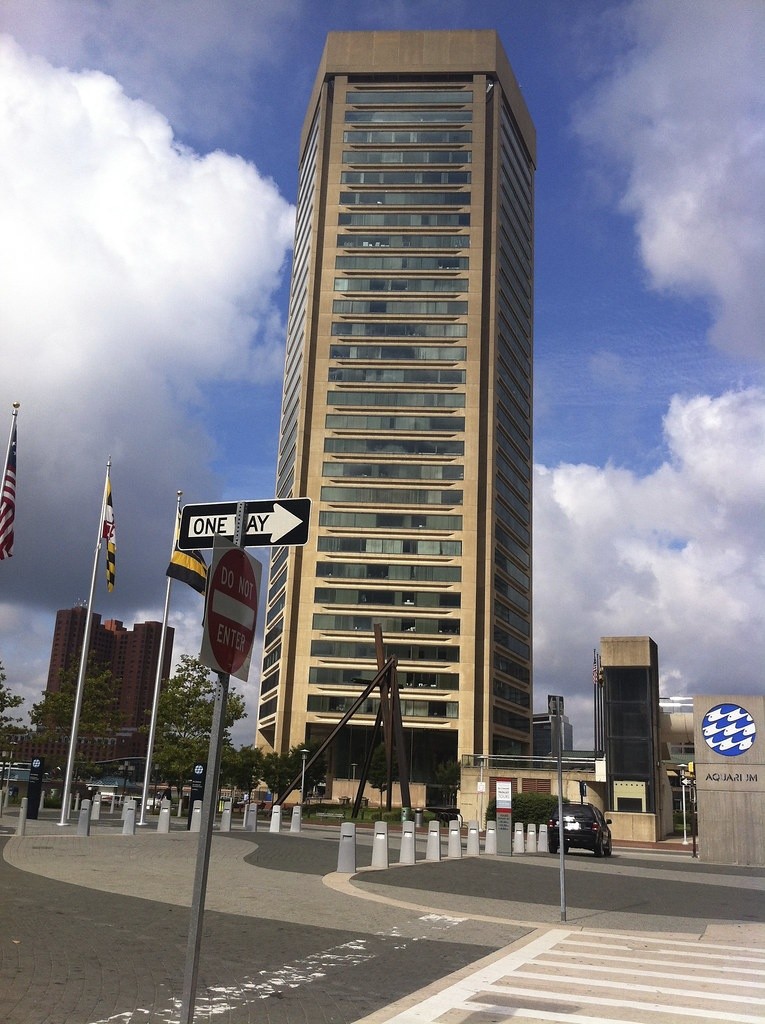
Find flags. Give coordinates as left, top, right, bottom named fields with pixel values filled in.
left=97, top=474, right=117, bottom=593
left=165, top=507, right=207, bottom=596
left=0, top=424, right=17, bottom=558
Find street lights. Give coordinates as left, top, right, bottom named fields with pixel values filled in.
left=123, top=759, right=131, bottom=799
left=152, top=764, right=161, bottom=813
left=1, top=741, right=18, bottom=808
left=299, top=748, right=310, bottom=807
left=677, top=763, right=689, bottom=845
left=350, top=763, right=359, bottom=804
left=476, top=755, right=489, bottom=830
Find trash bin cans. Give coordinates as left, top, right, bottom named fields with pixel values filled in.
left=415, top=808, right=423, bottom=827
left=401, top=807, right=410, bottom=824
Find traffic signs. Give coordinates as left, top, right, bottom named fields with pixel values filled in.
left=177, top=497, right=313, bottom=551
left=679, top=777, right=698, bottom=787
left=547, top=695, right=564, bottom=717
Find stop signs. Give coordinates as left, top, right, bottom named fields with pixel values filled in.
left=205, top=548, right=258, bottom=673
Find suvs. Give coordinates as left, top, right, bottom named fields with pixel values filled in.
left=545, top=801, right=613, bottom=858
